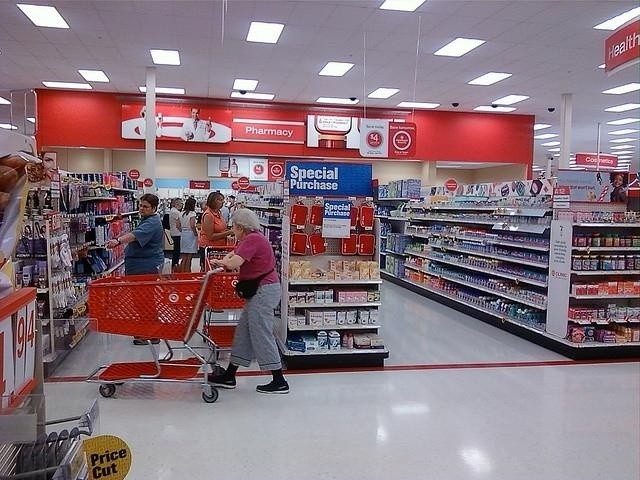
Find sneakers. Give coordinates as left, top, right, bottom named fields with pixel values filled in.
left=206, top=372, right=237, bottom=390
left=132, top=338, right=162, bottom=346
left=255, top=380, right=290, bottom=395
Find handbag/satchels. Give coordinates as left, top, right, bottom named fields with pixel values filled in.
left=234, top=267, right=277, bottom=301
left=161, top=213, right=172, bottom=230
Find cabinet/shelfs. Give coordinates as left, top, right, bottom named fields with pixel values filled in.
left=245, top=196, right=640, bottom=371
left=13, top=170, right=137, bottom=380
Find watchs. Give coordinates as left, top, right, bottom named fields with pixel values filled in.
left=114, top=238, right=122, bottom=244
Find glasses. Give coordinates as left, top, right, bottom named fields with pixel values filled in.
left=137, top=204, right=153, bottom=208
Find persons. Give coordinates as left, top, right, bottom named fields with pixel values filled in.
left=168, top=196, right=184, bottom=268
left=178, top=105, right=214, bottom=143
left=609, top=172, right=628, bottom=203
left=138, top=105, right=165, bottom=138
left=199, top=191, right=233, bottom=274
left=207, top=209, right=290, bottom=395
left=175, top=197, right=198, bottom=273
left=102, top=193, right=164, bottom=345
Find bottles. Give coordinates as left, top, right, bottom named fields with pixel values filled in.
left=43, top=310, right=76, bottom=350
left=237, top=190, right=270, bottom=207
left=343, top=333, right=353, bottom=349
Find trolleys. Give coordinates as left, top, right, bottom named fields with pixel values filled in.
left=204, top=245, right=245, bottom=359
left=86, top=267, right=225, bottom=404
